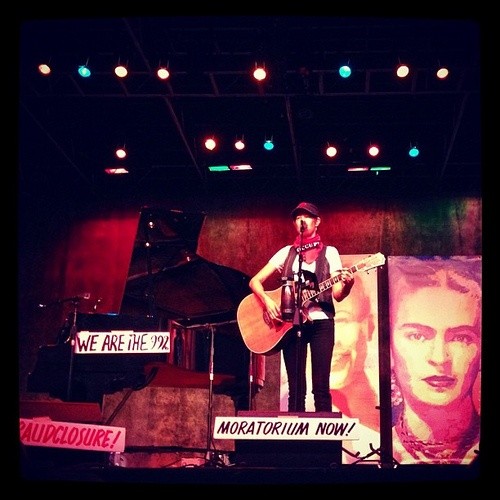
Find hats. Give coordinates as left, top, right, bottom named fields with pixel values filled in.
left=290, top=201, right=321, bottom=218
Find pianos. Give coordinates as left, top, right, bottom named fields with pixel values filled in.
left=27, top=205, right=266, bottom=411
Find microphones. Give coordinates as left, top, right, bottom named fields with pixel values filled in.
left=300, top=220, right=305, bottom=232
left=71, top=294, right=90, bottom=301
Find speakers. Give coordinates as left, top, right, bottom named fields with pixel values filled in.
left=19, top=421, right=111, bottom=471
left=234, top=410, right=343, bottom=468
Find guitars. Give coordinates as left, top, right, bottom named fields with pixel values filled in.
left=236, top=252, right=386, bottom=355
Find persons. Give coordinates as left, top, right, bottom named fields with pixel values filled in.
left=249, top=203, right=355, bottom=412
